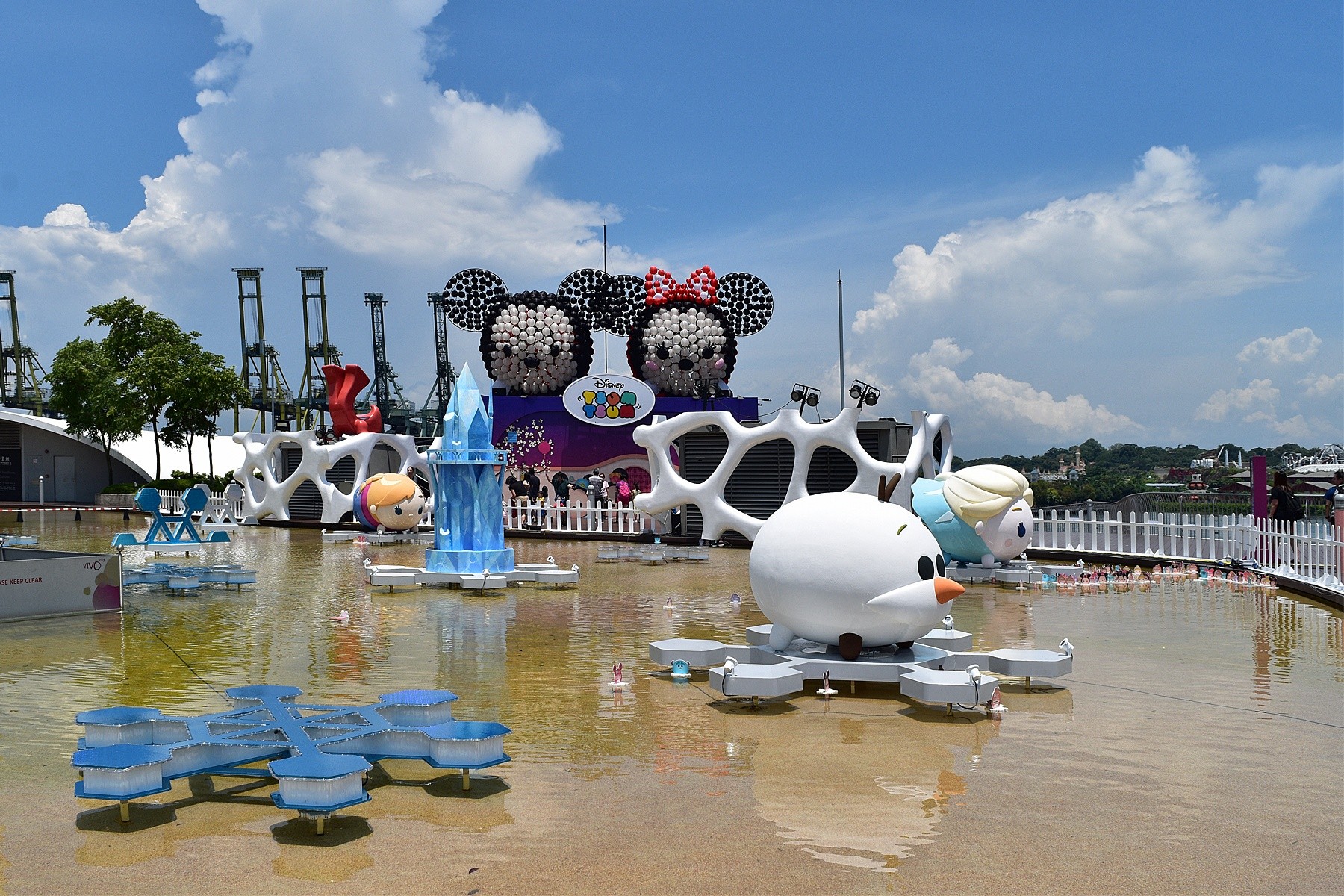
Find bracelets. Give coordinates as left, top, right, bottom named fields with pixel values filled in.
left=1269, top=517, right=1272, bottom=519
left=1325, top=516, right=1330, bottom=519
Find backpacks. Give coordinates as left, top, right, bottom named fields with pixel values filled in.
left=1276, top=484, right=1305, bottom=522
left=617, top=482, right=630, bottom=502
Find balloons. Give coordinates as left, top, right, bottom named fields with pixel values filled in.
left=441, top=266, right=775, bottom=401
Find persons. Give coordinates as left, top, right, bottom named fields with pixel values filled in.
left=1269, top=470, right=1294, bottom=567
left=406, top=466, right=419, bottom=534
left=1325, top=471, right=1344, bottom=573
left=504, top=464, right=643, bottom=531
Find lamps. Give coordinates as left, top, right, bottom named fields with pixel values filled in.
left=547, top=556, right=555, bottom=565
left=320, top=528, right=326, bottom=534
left=849, top=385, right=861, bottom=399
left=790, top=390, right=803, bottom=402
left=571, top=563, right=580, bottom=572
left=736, top=395, right=744, bottom=400
left=758, top=398, right=772, bottom=402
left=965, top=664, right=981, bottom=686
left=942, top=615, right=955, bottom=631
left=1059, top=638, right=1074, bottom=656
left=0, top=537, right=5, bottom=546
left=865, top=392, right=878, bottom=407
left=1077, top=559, right=1085, bottom=568
left=482, top=569, right=491, bottom=578
left=525, top=523, right=542, bottom=531
left=1026, top=564, right=1034, bottom=573
left=1020, top=552, right=1027, bottom=561
left=377, top=530, right=383, bottom=536
left=723, top=656, right=739, bottom=676
left=117, top=544, right=124, bottom=553
left=693, top=396, right=700, bottom=401
left=370, top=566, right=380, bottom=575
left=362, top=557, right=372, bottom=567
left=806, top=392, right=818, bottom=407
left=521, top=395, right=528, bottom=399
